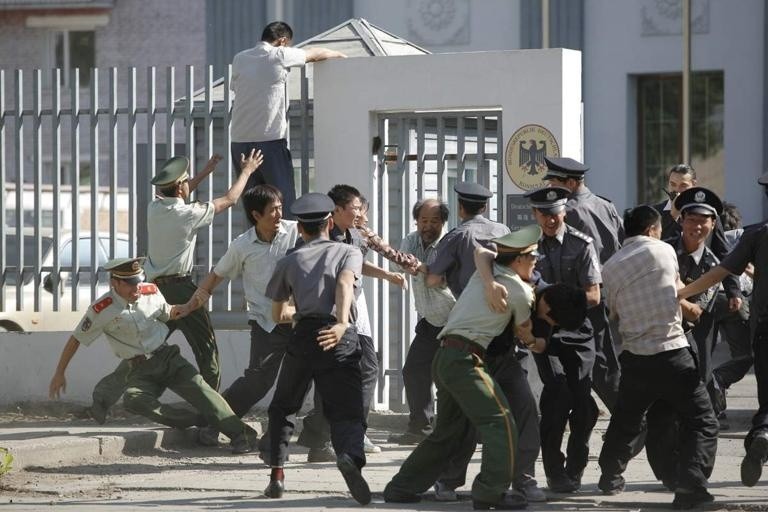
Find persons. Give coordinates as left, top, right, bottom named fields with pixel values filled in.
left=88, top=149, right=264, bottom=424
left=49, top=257, right=257, bottom=454
left=353, top=195, right=424, bottom=290
left=264, top=194, right=371, bottom=506
left=389, top=199, right=458, bottom=445
left=230, top=22, right=347, bottom=221
left=705, top=200, right=753, bottom=420
left=652, top=164, right=697, bottom=239
left=171, top=185, right=338, bottom=462
left=677, top=172, right=768, bottom=486
left=383, top=225, right=542, bottom=508
left=541, top=157, right=646, bottom=458
left=522, top=185, right=602, bottom=493
left=286, top=185, right=365, bottom=252
left=597, top=205, right=714, bottom=511
left=647, top=187, right=720, bottom=490
left=433, top=245, right=588, bottom=503
left=426, top=182, right=529, bottom=379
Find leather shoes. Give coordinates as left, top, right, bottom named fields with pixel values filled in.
left=544, top=464, right=581, bottom=493
left=381, top=488, right=421, bottom=502
left=566, top=462, right=584, bottom=481
left=387, top=433, right=417, bottom=445
left=471, top=492, right=529, bottom=512
left=336, top=453, right=373, bottom=506
left=262, top=478, right=285, bottom=499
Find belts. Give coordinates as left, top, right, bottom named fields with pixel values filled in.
left=442, top=337, right=487, bottom=359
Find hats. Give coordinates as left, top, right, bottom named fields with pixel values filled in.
left=101, top=255, right=149, bottom=285
left=450, top=181, right=496, bottom=205
left=489, top=223, right=543, bottom=257
left=150, top=155, right=190, bottom=190
left=673, top=185, right=723, bottom=219
left=523, top=186, right=573, bottom=216
left=541, top=156, right=591, bottom=182
left=290, top=192, right=337, bottom=223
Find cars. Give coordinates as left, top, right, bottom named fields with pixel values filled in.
left=0, top=226, right=137, bottom=332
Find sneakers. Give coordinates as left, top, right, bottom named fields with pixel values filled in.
left=200, top=424, right=217, bottom=444
left=307, top=445, right=338, bottom=463
left=668, top=492, right=716, bottom=510
left=509, top=476, right=548, bottom=503
left=230, top=429, right=253, bottom=454
left=435, top=479, right=459, bottom=505
left=740, top=434, right=768, bottom=489
left=359, top=433, right=382, bottom=454
left=597, top=475, right=628, bottom=490
